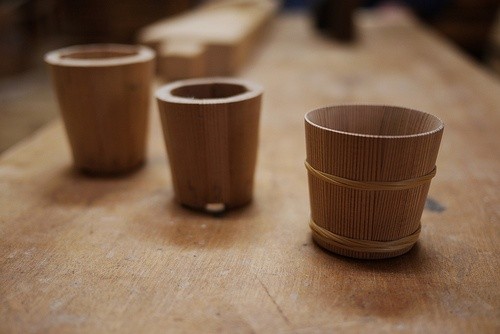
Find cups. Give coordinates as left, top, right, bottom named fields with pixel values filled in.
left=155, top=78, right=263, bottom=215
left=305, top=104, right=444, bottom=260
left=46, top=45, right=155, bottom=177
